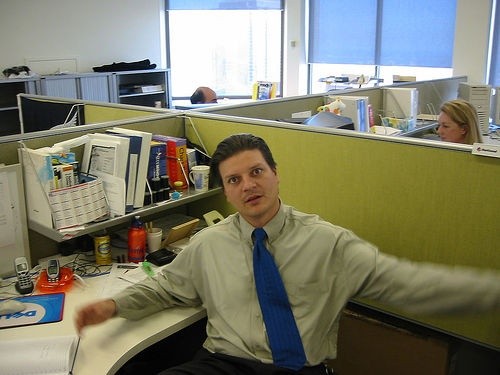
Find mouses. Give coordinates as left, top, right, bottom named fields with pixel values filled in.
left=0, top=299, right=24, bottom=315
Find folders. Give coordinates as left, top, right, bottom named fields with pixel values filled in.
left=329, top=95, right=370, bottom=133
left=101, top=126, right=152, bottom=213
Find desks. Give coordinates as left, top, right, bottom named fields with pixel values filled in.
left=374, top=114, right=440, bottom=137
left=0, top=218, right=208, bottom=375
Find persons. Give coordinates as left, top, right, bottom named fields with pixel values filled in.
left=73, top=134, right=500, bottom=375
left=438, top=99, right=484, bottom=145
left=190, top=87, right=218, bottom=104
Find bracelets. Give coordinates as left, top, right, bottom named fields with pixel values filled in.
left=103, top=300, right=116, bottom=318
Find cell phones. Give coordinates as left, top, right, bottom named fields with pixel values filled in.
left=46, top=259, right=59, bottom=282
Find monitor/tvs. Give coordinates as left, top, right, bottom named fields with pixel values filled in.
left=303, top=110, right=354, bottom=130
left=1, top=163, right=33, bottom=285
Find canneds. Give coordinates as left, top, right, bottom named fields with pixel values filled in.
left=94, top=233, right=112, bottom=265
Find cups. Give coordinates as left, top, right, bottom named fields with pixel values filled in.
left=154, top=101, right=161, bottom=107
left=94, top=233, right=112, bottom=265
left=188, top=165, right=210, bottom=192
left=146, top=228, right=162, bottom=252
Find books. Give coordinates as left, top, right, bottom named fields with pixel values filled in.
left=368, top=104, right=376, bottom=134
left=48, top=152, right=79, bottom=192
left=0, top=336, right=81, bottom=375
left=259, top=82, right=276, bottom=100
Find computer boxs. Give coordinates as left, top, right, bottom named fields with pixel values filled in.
left=383, top=86, right=419, bottom=118
left=325, top=95, right=369, bottom=133
left=457, top=82, right=492, bottom=135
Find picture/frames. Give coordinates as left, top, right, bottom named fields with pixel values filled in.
left=25, top=56, right=80, bottom=95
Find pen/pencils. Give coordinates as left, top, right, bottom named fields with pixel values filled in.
left=145, top=221, right=153, bottom=232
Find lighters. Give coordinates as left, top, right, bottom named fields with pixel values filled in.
left=143, top=262, right=154, bottom=275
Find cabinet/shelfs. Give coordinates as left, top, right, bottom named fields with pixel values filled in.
left=41, top=68, right=172, bottom=109
left=317, top=74, right=384, bottom=93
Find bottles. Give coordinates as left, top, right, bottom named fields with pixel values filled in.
left=127, top=216, right=146, bottom=263
left=173, top=181, right=183, bottom=196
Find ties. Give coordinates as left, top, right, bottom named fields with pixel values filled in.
left=253, top=227, right=306, bottom=371
